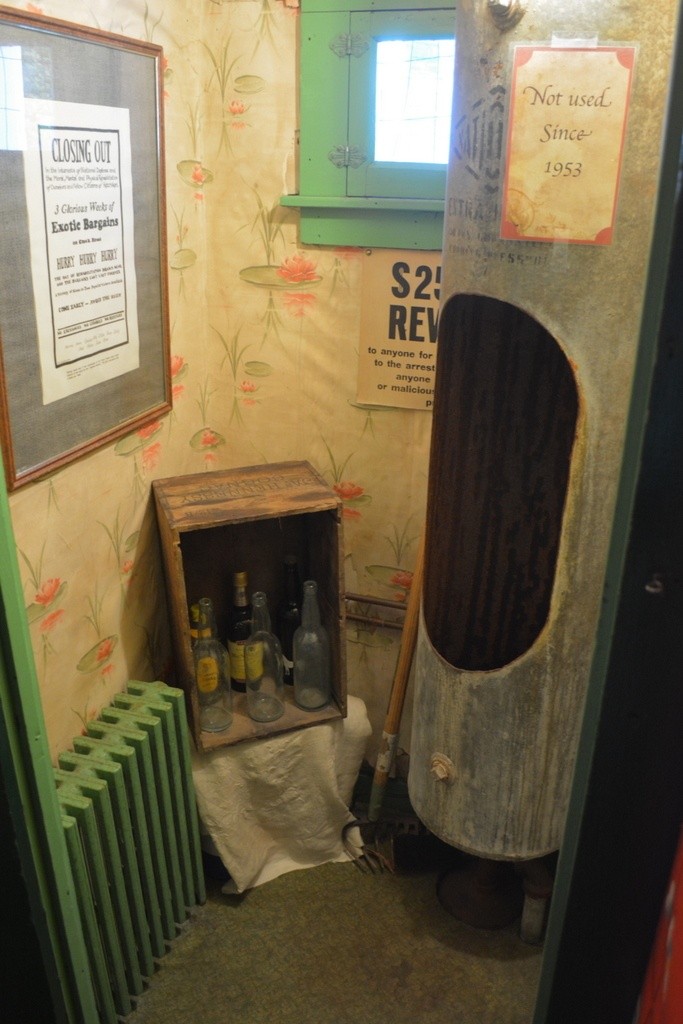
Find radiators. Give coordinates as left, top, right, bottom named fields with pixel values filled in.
left=48, top=678, right=211, bottom=1018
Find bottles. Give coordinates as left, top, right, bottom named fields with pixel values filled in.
left=292, top=580, right=333, bottom=712
left=224, top=569, right=265, bottom=693
left=188, top=603, right=223, bottom=706
left=192, top=597, right=233, bottom=733
left=243, top=592, right=286, bottom=723
left=279, top=555, right=302, bottom=686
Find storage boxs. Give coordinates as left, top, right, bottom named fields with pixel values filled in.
left=153, top=457, right=348, bottom=755
